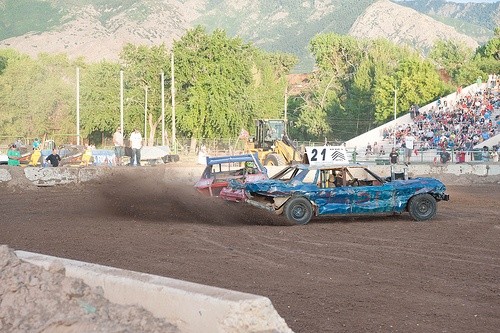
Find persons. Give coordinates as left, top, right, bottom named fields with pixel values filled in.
left=334, top=172, right=351, bottom=187
left=353, top=148, right=358, bottom=164
left=15, top=137, right=24, bottom=151
left=45, top=149, right=62, bottom=167
left=7, top=145, right=21, bottom=166
left=365, top=73, right=500, bottom=166
left=32, top=138, right=40, bottom=150
left=130, top=127, right=142, bottom=166
left=113, top=126, right=123, bottom=165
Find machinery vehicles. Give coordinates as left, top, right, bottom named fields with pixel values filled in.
left=239, top=119, right=303, bottom=172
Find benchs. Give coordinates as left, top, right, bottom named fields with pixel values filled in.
left=351, top=74, right=499, bottom=162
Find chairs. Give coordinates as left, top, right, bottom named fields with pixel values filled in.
left=327, top=174, right=336, bottom=188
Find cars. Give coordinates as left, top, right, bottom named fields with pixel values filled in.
left=0, top=138, right=179, bottom=167
left=245, top=163, right=449, bottom=225
left=193, top=154, right=269, bottom=203
